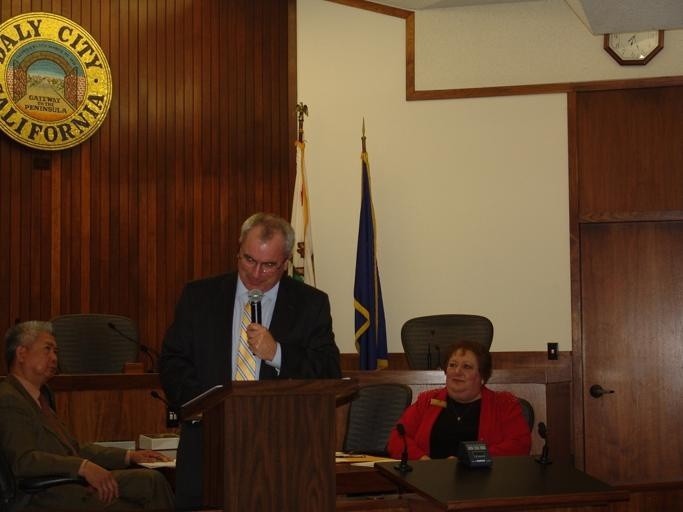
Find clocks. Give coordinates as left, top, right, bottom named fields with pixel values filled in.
left=603, top=29, right=665, bottom=66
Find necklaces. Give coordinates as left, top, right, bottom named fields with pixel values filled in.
left=450, top=400, right=472, bottom=421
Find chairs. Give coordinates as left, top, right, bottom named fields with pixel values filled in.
left=342, top=382, right=413, bottom=457
left=400, top=313, right=494, bottom=377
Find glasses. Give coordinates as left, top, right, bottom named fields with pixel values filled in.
left=233, top=247, right=287, bottom=274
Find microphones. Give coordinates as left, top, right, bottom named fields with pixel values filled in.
left=430, top=329, right=444, bottom=369
left=151, top=391, right=182, bottom=427
left=535, top=422, right=552, bottom=464
left=394, top=424, right=413, bottom=472
left=248, top=288, right=263, bottom=356
left=107, top=322, right=160, bottom=373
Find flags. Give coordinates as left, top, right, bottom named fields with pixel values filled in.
left=286, top=131, right=317, bottom=290
left=351, top=149, right=390, bottom=371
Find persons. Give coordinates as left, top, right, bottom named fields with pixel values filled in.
left=387, top=337, right=535, bottom=463
left=0, top=320, right=176, bottom=510
left=157, top=211, right=341, bottom=510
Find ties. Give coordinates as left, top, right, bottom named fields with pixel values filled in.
left=38, top=393, right=67, bottom=444
left=235, top=301, right=256, bottom=382
